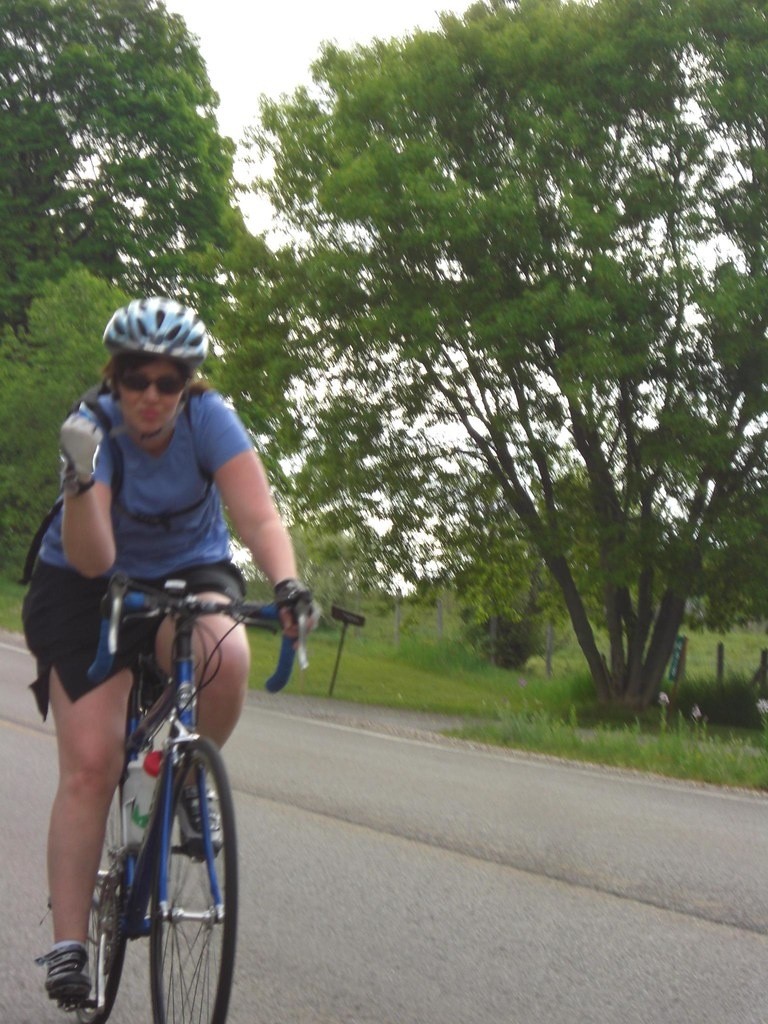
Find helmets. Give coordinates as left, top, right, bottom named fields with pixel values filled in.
left=102, top=296, right=209, bottom=377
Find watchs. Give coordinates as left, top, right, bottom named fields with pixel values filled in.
left=59, top=473, right=96, bottom=498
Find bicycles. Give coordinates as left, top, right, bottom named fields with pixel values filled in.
left=43, top=568, right=316, bottom=1023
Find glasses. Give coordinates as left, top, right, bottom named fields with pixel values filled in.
left=118, top=370, right=188, bottom=393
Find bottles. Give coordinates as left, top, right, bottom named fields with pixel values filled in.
left=127, top=749, right=163, bottom=844
left=122, top=757, right=145, bottom=845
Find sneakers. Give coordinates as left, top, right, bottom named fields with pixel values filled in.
left=176, top=784, right=223, bottom=858
left=33, top=943, right=92, bottom=999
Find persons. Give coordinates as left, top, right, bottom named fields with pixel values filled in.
left=23, top=298, right=326, bottom=1001
